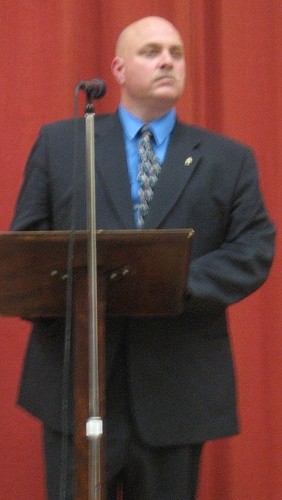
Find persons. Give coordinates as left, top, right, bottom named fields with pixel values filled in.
left=9, top=15, right=276, bottom=500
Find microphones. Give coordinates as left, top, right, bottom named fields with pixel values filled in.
left=77, top=78, right=106, bottom=99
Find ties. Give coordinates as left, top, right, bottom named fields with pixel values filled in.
left=136, top=127, right=164, bottom=229
